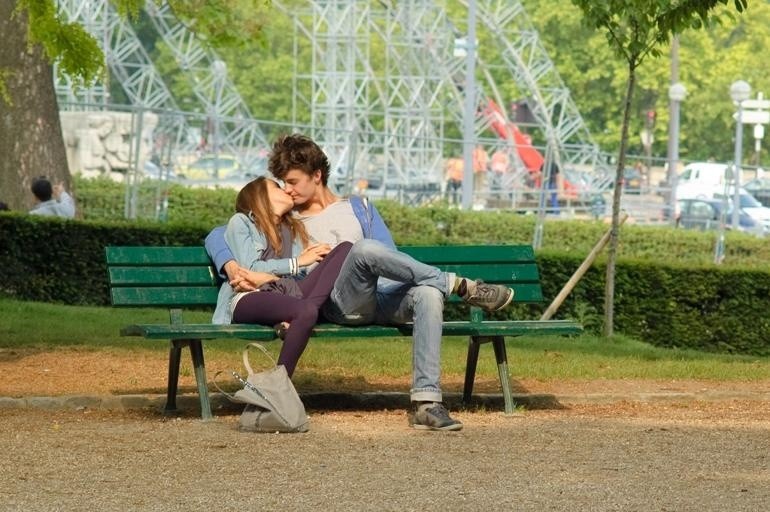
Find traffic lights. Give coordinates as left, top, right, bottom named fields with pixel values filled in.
left=641, top=110, right=657, bottom=128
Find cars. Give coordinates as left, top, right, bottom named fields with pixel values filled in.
left=658, top=162, right=770, bottom=235
left=354, top=166, right=443, bottom=192
left=472, top=167, right=605, bottom=219
left=175, top=155, right=240, bottom=181
left=609, top=168, right=642, bottom=194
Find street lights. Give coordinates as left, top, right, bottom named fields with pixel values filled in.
left=731, top=80, right=751, bottom=230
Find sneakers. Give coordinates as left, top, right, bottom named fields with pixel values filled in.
left=465, top=278, right=515, bottom=310
left=275, top=322, right=289, bottom=340
left=412, top=404, right=463, bottom=430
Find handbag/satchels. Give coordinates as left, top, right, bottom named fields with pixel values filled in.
left=213, top=344, right=310, bottom=433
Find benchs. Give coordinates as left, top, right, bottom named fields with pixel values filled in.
left=104, top=242, right=588, bottom=421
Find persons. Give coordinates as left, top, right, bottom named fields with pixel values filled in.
left=472, top=143, right=489, bottom=198
left=203, top=132, right=515, bottom=431
left=28, top=179, right=75, bottom=219
left=209, top=176, right=353, bottom=378
left=491, top=144, right=512, bottom=198
left=442, top=150, right=465, bottom=205
